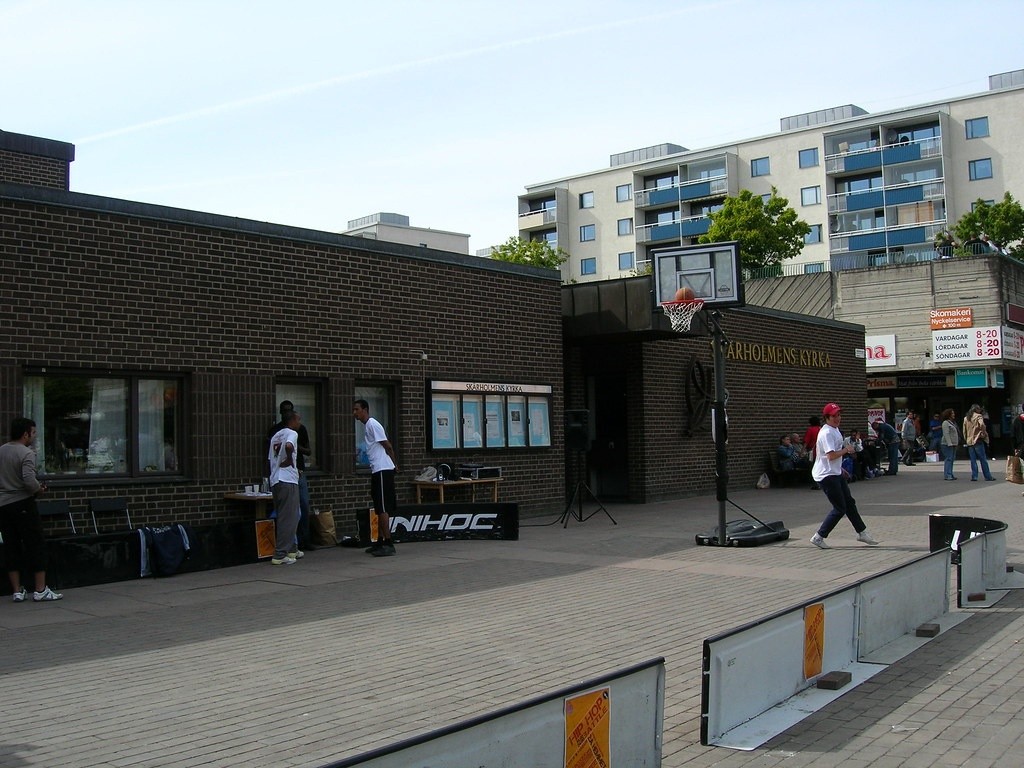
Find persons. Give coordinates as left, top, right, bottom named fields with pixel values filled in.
left=264, top=400, right=315, bottom=565
left=1011, top=404, right=1024, bottom=461
left=963, top=404, right=996, bottom=481
left=353, top=399, right=400, bottom=556
left=777, top=416, right=885, bottom=490
left=940, top=408, right=962, bottom=481
left=0, top=416, right=63, bottom=601
left=809, top=401, right=884, bottom=549
left=871, top=421, right=901, bottom=476
left=914, top=413, right=921, bottom=437
left=929, top=413, right=943, bottom=451
left=901, top=410, right=916, bottom=466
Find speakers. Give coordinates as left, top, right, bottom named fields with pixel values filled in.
left=564, top=410, right=594, bottom=451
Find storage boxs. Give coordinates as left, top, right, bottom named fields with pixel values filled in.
left=458, top=467, right=502, bottom=479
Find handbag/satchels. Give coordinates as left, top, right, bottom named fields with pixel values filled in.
left=307, top=505, right=338, bottom=545
left=757, top=472, right=770, bottom=489
left=1005, top=455, right=1024, bottom=484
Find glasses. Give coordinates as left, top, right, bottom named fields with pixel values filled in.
left=934, top=416, right=940, bottom=417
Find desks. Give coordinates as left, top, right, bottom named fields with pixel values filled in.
left=226, top=492, right=272, bottom=519
left=413, top=480, right=504, bottom=502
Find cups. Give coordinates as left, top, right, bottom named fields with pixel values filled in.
left=254, top=485, right=260, bottom=493
left=245, top=486, right=252, bottom=494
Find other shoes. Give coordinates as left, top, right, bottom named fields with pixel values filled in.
left=971, top=478, right=979, bottom=481
left=900, top=459, right=917, bottom=466
left=857, top=531, right=879, bottom=545
left=986, top=478, right=996, bottom=481
left=810, top=486, right=821, bottom=490
left=882, top=471, right=897, bottom=476
left=874, top=468, right=879, bottom=473
left=812, top=538, right=831, bottom=549
left=944, top=476, right=957, bottom=480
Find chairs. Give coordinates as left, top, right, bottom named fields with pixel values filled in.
left=91, top=499, right=133, bottom=533
left=38, top=502, right=76, bottom=537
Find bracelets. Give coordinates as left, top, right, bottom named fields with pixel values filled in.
left=395, top=464, right=398, bottom=467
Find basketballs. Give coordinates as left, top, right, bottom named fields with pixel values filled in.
left=674, top=287, right=695, bottom=304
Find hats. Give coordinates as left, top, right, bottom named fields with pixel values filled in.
left=823, top=403, right=842, bottom=417
left=279, top=401, right=293, bottom=411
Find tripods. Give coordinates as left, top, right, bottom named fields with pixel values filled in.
left=561, top=450, right=618, bottom=529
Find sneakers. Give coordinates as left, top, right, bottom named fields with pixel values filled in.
left=33, top=585, right=64, bottom=601
left=288, top=550, right=304, bottom=558
left=272, top=556, right=297, bottom=566
left=13, top=586, right=28, bottom=602
left=365, top=540, right=395, bottom=556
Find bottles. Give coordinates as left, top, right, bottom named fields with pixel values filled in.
left=263, top=478, right=270, bottom=492
